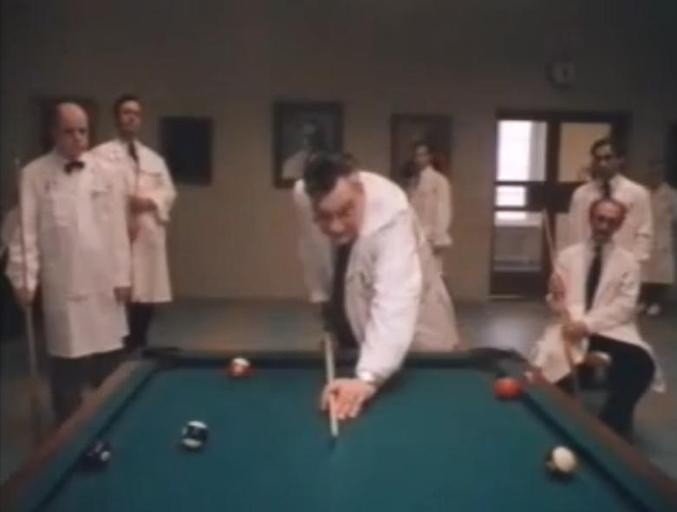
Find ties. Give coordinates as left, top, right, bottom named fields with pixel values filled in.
left=583, top=241, right=604, bottom=314
left=127, top=138, right=140, bottom=163
left=602, top=180, right=612, bottom=199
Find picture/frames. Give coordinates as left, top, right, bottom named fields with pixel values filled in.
left=384, top=111, right=456, bottom=196
left=270, top=98, right=343, bottom=192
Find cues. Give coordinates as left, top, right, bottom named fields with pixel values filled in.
left=541, top=208, right=581, bottom=404
left=12, top=158, right=42, bottom=442
left=322, top=331, right=340, bottom=436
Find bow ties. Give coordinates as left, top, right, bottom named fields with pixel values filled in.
left=62, top=159, right=86, bottom=174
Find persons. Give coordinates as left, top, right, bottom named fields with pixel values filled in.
left=1, top=98, right=134, bottom=427
left=565, top=136, right=655, bottom=265
left=529, top=195, right=668, bottom=446
left=632, top=160, right=677, bottom=318
left=88, top=92, right=178, bottom=389
left=398, top=140, right=453, bottom=279
left=287, top=142, right=461, bottom=422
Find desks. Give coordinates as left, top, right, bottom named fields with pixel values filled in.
left=0, top=345, right=675, bottom=511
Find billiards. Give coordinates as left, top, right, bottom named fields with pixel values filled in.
left=84, top=439, right=112, bottom=466
left=546, top=446, right=579, bottom=476
left=229, top=357, right=249, bottom=378
left=180, top=420, right=208, bottom=446
left=494, top=377, right=520, bottom=397
